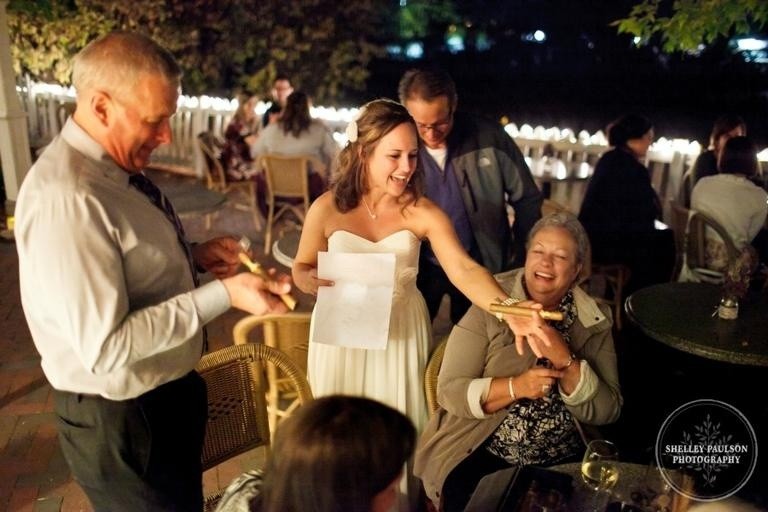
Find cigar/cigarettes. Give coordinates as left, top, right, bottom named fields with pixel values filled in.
left=490, top=303, right=565, bottom=323
left=236, top=252, right=298, bottom=309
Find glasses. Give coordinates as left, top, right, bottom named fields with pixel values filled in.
left=404, top=106, right=456, bottom=133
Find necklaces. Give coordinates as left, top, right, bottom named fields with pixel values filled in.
left=363, top=196, right=394, bottom=222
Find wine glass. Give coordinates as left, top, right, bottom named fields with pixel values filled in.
left=580, top=439, right=622, bottom=512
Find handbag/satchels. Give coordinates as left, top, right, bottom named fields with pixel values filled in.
left=675, top=209, right=734, bottom=292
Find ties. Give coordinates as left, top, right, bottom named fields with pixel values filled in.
left=128, top=174, right=209, bottom=357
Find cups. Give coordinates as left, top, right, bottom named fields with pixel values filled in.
left=642, top=446, right=684, bottom=494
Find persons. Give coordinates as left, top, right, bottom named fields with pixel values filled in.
left=215, top=394, right=417, bottom=512
left=293, top=98, right=551, bottom=503
left=414, top=212, right=624, bottom=511
left=220, top=92, right=276, bottom=219
left=251, top=91, right=341, bottom=225
left=399, top=67, right=544, bottom=324
left=690, top=119, right=762, bottom=193
left=263, top=74, right=293, bottom=127
left=578, top=115, right=675, bottom=298
left=682, top=136, right=768, bottom=284
left=14, top=32, right=294, bottom=510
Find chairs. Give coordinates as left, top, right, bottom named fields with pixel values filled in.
left=177, top=126, right=767, bottom=512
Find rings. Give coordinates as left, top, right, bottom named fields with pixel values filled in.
left=542, top=382, right=550, bottom=396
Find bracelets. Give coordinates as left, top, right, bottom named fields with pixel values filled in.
left=495, top=299, right=520, bottom=323
left=508, top=375, right=516, bottom=400
left=557, top=352, right=575, bottom=372
